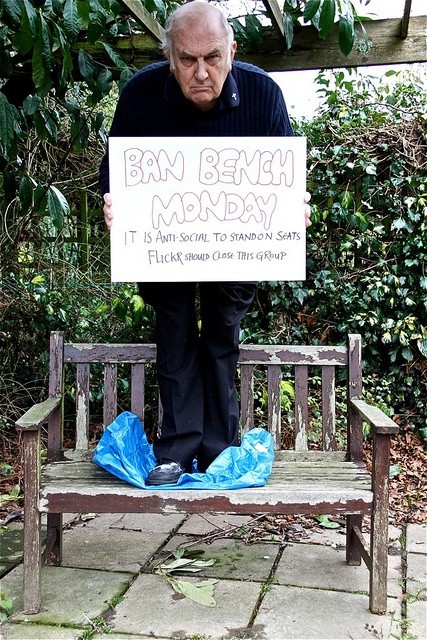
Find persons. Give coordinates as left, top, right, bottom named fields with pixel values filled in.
left=98, top=1, right=311, bottom=485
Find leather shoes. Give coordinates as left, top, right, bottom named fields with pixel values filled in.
left=145, top=459, right=190, bottom=485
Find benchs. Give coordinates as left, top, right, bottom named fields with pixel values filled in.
left=15, top=333, right=398, bottom=614
